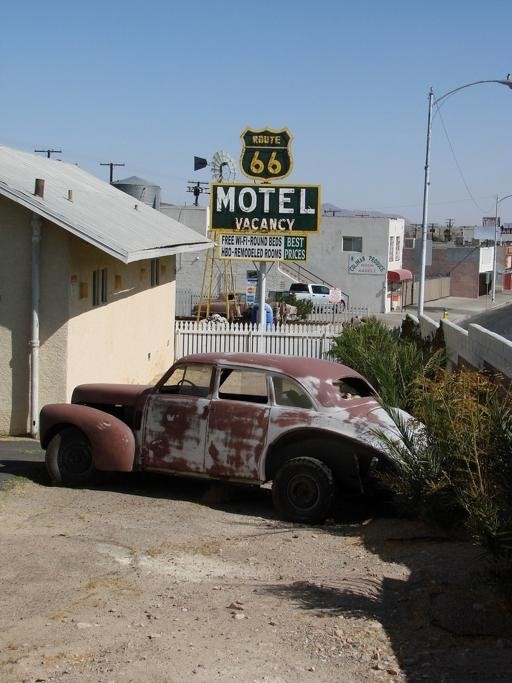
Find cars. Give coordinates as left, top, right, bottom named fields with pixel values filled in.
left=192, top=292, right=300, bottom=322
left=38, top=351, right=434, bottom=525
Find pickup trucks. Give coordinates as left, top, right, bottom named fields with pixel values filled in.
left=267, top=282, right=346, bottom=314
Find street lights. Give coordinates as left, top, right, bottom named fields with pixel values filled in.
left=417, top=79, right=512, bottom=317
